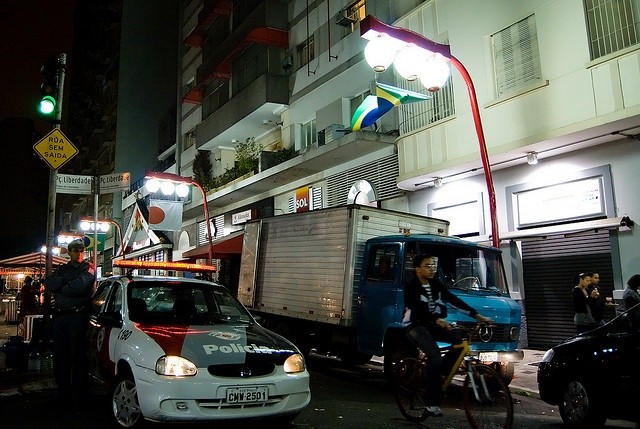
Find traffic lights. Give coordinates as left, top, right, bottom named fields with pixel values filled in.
left=38, top=53, right=61, bottom=117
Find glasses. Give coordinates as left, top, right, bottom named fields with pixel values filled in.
left=419, top=264, right=435, bottom=268
left=70, top=249, right=84, bottom=253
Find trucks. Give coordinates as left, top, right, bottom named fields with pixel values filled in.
left=236, top=203, right=524, bottom=395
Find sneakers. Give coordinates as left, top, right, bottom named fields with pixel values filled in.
left=426, top=406, right=442, bottom=417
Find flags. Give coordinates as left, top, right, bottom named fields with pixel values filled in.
left=349, top=82, right=433, bottom=132
left=150, top=200, right=184, bottom=233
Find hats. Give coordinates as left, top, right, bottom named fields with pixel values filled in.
left=68, top=239, right=85, bottom=248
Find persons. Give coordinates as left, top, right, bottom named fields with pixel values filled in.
left=401, top=254, right=492, bottom=417
left=45, top=238, right=97, bottom=399
left=20, top=276, right=41, bottom=312
left=587, top=272, right=605, bottom=325
left=573, top=273, right=596, bottom=334
left=622, top=274, right=640, bottom=310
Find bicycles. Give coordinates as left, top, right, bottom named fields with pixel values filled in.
left=390, top=318, right=513, bottom=427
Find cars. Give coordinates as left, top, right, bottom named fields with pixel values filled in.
left=537, top=302, right=640, bottom=427
left=87, top=259, right=311, bottom=429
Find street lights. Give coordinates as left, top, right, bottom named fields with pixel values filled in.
left=145, top=169, right=213, bottom=282
left=360, top=14, right=503, bottom=296
left=58, top=233, right=93, bottom=263
left=81, top=215, right=125, bottom=275
